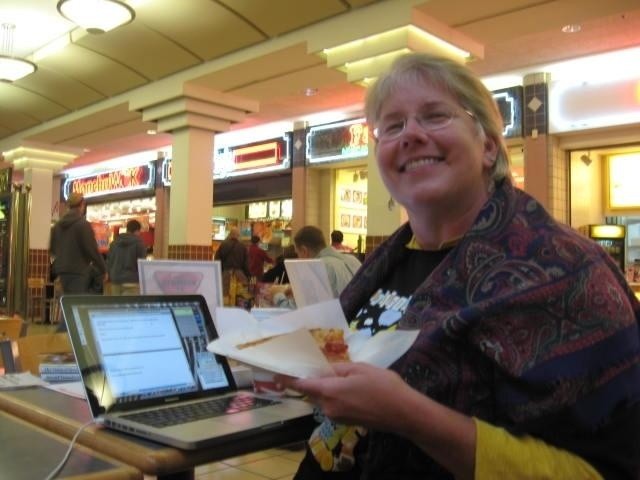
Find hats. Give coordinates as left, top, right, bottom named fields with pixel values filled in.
left=67, top=191, right=83, bottom=206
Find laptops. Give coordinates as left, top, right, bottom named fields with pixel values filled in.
left=60, top=293, right=316, bottom=453
left=284, top=256, right=334, bottom=308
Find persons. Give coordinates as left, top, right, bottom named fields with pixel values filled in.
left=286, top=50, right=639, bottom=478
left=46, top=191, right=155, bottom=332
left=215, top=224, right=362, bottom=312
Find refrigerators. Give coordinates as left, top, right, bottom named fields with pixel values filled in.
left=580, top=223, right=627, bottom=274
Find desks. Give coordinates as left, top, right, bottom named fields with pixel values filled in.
left=0, top=376, right=319, bottom=480
left=0, top=411, right=144, bottom=480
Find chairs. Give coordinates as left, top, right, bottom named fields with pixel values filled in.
left=26, top=277, right=45, bottom=324
left=0, top=333, right=74, bottom=376
left=0, top=317, right=23, bottom=369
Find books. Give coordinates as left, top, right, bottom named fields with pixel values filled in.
left=37, top=350, right=81, bottom=383
left=1, top=370, right=40, bottom=391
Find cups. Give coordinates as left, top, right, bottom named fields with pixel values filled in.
left=248, top=307, right=292, bottom=398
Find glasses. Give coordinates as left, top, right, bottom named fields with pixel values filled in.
left=367, top=100, right=486, bottom=143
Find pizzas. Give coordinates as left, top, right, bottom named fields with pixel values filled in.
left=237, top=327, right=350, bottom=363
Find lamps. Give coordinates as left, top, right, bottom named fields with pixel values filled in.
left=57, top=0, right=136, bottom=34
left=0, top=23, right=37, bottom=83
left=579, top=148, right=592, bottom=166
